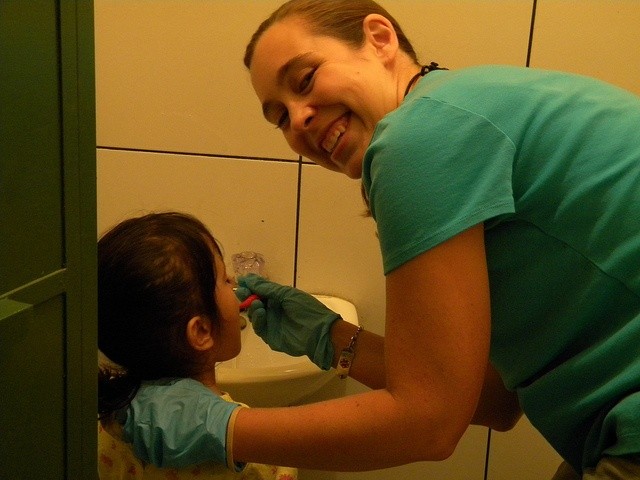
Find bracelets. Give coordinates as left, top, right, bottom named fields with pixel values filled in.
left=336, top=325, right=363, bottom=379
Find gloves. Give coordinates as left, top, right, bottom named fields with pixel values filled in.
left=118, top=376, right=248, bottom=475
left=236, top=271, right=343, bottom=373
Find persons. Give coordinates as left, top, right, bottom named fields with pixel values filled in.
left=98, top=211, right=299, bottom=480
left=103, top=0, right=640, bottom=480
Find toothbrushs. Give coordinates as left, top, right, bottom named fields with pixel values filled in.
left=238, top=294, right=262, bottom=311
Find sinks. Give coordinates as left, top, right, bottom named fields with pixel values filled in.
left=214, top=294, right=359, bottom=408
